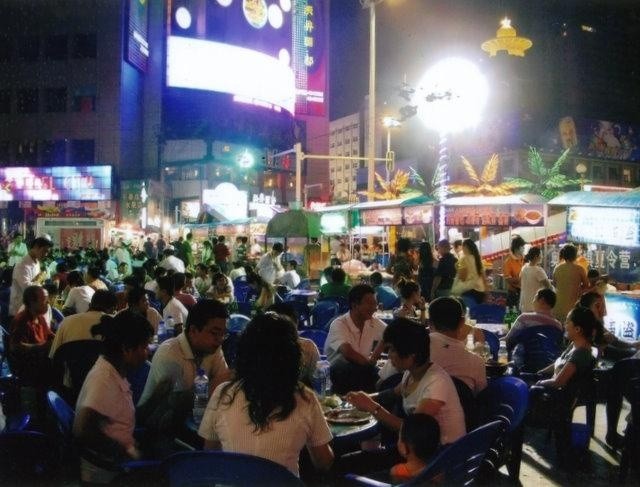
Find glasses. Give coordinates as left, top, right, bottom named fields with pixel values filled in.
left=202, top=326, right=230, bottom=341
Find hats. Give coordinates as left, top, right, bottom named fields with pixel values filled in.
left=274, top=243, right=284, bottom=252
left=122, top=238, right=133, bottom=246
left=163, top=244, right=174, bottom=251
left=35, top=231, right=52, bottom=241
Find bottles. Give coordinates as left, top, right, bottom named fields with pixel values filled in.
left=498, top=341, right=510, bottom=364
left=166, top=316, right=175, bottom=338
left=193, top=369, right=209, bottom=425
left=157, top=321, right=167, bottom=345
left=312, top=364, right=326, bottom=401
left=319, top=355, right=333, bottom=396
left=503, top=305, right=519, bottom=331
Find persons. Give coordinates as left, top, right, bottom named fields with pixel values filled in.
left=0, top=226, right=639, bottom=486
left=137, top=194, right=171, bottom=228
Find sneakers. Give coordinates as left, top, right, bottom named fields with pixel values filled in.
left=605, top=432, right=624, bottom=450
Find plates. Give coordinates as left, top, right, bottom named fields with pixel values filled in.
left=323, top=408, right=373, bottom=423
